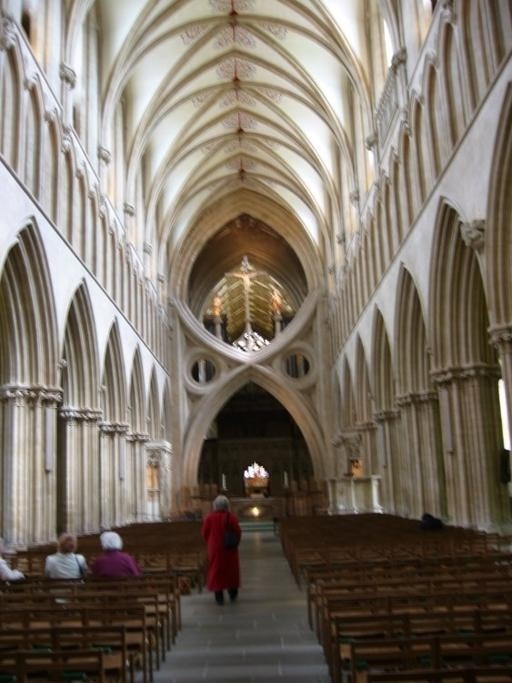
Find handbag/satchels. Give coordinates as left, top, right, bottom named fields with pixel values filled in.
left=223, top=512, right=238, bottom=548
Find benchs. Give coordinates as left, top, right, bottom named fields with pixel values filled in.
left=2, top=518, right=207, bottom=681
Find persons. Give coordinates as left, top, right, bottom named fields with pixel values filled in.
left=90, top=530, right=147, bottom=615
left=418, top=513, right=442, bottom=531
left=43, top=532, right=94, bottom=618
left=0, top=537, right=25, bottom=584
left=200, top=493, right=243, bottom=607
